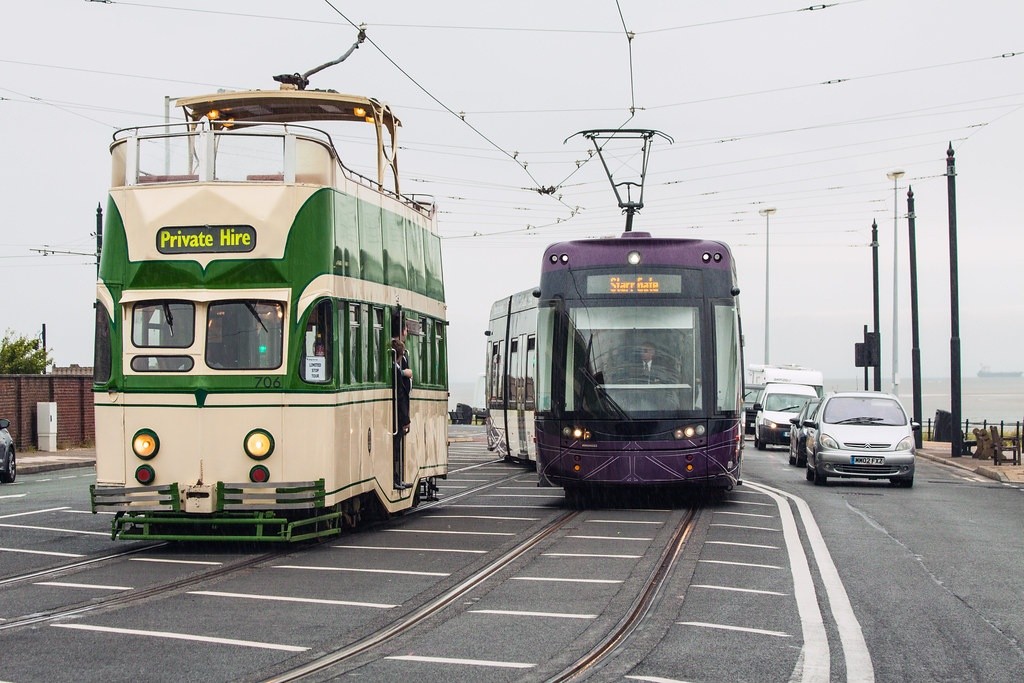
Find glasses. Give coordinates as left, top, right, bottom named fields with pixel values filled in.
left=640, top=349, right=649, bottom=353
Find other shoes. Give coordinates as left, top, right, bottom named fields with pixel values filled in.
left=394, top=483, right=406, bottom=490
left=396, top=477, right=413, bottom=488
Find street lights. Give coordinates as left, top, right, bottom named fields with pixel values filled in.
left=759, top=205, right=776, bottom=366
left=886, top=169, right=905, bottom=396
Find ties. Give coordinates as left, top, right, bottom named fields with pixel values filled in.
left=645, top=363, right=648, bottom=372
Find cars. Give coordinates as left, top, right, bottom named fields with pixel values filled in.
left=0, top=418, right=16, bottom=482
left=802, top=391, right=920, bottom=488
left=789, top=398, right=822, bottom=468
left=746, top=384, right=766, bottom=434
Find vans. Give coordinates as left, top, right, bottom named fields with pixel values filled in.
left=753, top=384, right=819, bottom=450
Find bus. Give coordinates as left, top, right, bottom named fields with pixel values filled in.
left=484, top=232, right=745, bottom=501
left=90, top=73, right=450, bottom=541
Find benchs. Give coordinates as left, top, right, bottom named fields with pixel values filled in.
left=961, top=425, right=1021, bottom=466
left=448, top=412, right=471, bottom=425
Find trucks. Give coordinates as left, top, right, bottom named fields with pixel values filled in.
left=748, top=365, right=823, bottom=399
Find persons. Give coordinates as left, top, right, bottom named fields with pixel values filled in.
left=638, top=341, right=657, bottom=386
left=393, top=316, right=413, bottom=490
